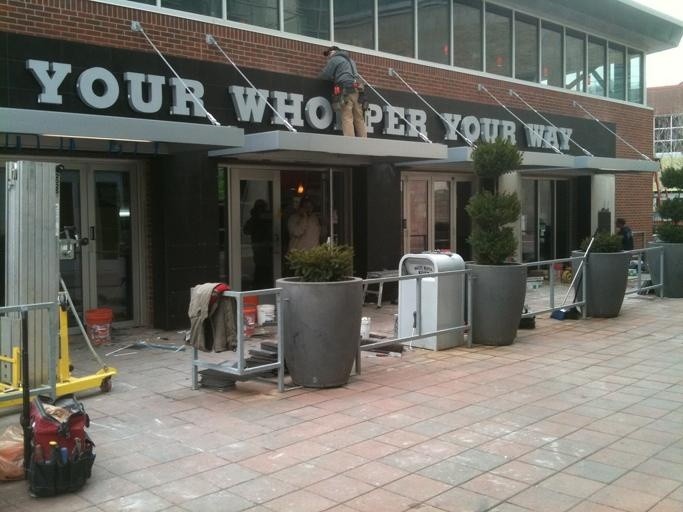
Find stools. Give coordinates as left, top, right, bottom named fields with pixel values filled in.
left=364, top=269, right=397, bottom=308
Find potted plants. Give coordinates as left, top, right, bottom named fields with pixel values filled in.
left=276, top=238, right=364, bottom=387
left=573, top=228, right=632, bottom=317
left=465, top=134, right=527, bottom=345
left=647, top=167, right=683, bottom=298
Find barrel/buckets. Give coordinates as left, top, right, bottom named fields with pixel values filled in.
left=85, top=308, right=113, bottom=344
left=242, top=308, right=255, bottom=338
left=257, top=304, right=276, bottom=324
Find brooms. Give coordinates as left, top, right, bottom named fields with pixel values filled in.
left=549, top=237, right=595, bottom=319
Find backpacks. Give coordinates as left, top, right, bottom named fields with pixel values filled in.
left=28, top=392, right=96, bottom=498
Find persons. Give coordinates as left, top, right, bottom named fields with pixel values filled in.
left=285, top=195, right=321, bottom=250
left=244, top=199, right=272, bottom=288
left=317, top=46, right=367, bottom=138
left=612, top=219, right=634, bottom=251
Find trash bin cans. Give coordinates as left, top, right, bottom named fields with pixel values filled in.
left=398, top=253, right=465, bottom=351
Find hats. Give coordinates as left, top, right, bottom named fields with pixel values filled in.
left=323, top=45, right=341, bottom=55
